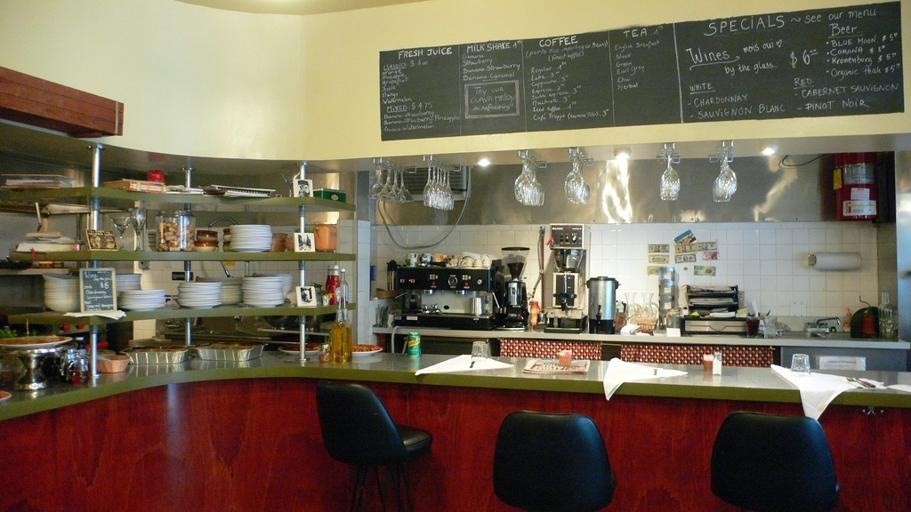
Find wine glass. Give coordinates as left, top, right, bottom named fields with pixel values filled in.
left=659, top=150, right=681, bottom=202
left=711, top=145, right=738, bottom=203
left=422, top=158, right=455, bottom=211
left=368, top=157, right=415, bottom=204
left=109, top=207, right=147, bottom=252
left=564, top=151, right=592, bottom=206
left=513, top=153, right=545, bottom=207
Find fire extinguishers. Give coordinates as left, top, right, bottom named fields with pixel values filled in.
left=833, top=152, right=880, bottom=221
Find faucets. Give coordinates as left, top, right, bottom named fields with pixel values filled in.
left=560, top=293, right=570, bottom=310
left=595, top=305, right=602, bottom=327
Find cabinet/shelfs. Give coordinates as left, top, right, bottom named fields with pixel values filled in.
left=0, top=160, right=358, bottom=400
left=369, top=323, right=910, bottom=373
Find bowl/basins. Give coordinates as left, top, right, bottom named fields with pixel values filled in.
left=194, top=228, right=230, bottom=252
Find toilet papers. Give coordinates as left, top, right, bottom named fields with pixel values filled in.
left=814, top=251, right=862, bottom=270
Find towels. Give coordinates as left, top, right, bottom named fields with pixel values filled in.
left=770, top=363, right=858, bottom=421
left=415, top=353, right=516, bottom=382
left=603, top=357, right=687, bottom=400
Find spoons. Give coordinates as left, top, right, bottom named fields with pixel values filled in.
left=847, top=375, right=876, bottom=389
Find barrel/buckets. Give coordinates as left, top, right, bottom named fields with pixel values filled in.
left=586, top=276, right=619, bottom=320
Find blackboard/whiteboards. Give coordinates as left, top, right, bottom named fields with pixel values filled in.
left=79, top=267, right=117, bottom=313
left=379, top=1, right=905, bottom=142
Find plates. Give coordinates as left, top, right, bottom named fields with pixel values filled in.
left=42, top=272, right=79, bottom=313
left=228, top=224, right=274, bottom=253
left=116, top=272, right=167, bottom=312
left=176, top=272, right=293, bottom=309
left=277, top=344, right=319, bottom=355
left=352, top=347, right=383, bottom=357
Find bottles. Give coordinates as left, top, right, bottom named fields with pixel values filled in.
left=878, top=291, right=896, bottom=339
left=328, top=286, right=352, bottom=365
left=319, top=343, right=331, bottom=363
left=155, top=208, right=196, bottom=251
left=60, top=347, right=89, bottom=385
left=712, top=352, right=723, bottom=376
left=325, top=264, right=350, bottom=305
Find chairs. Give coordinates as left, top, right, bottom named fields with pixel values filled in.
left=314, top=378, right=432, bottom=512
left=493, top=409, right=616, bottom=511
left=710, top=410, right=842, bottom=511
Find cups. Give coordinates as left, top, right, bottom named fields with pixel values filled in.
left=403, top=251, right=446, bottom=267
left=703, top=354, right=712, bottom=370
left=558, top=350, right=572, bottom=367
left=791, top=353, right=810, bottom=373
left=745, top=315, right=777, bottom=339
left=469, top=296, right=485, bottom=316
left=471, top=339, right=491, bottom=366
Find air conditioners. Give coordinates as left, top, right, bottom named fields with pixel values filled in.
left=383, top=166, right=471, bottom=204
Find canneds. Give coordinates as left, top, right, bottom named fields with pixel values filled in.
left=407, top=332, right=422, bottom=359
left=421, top=251, right=432, bottom=268
left=433, top=252, right=443, bottom=267
left=407, top=252, right=418, bottom=267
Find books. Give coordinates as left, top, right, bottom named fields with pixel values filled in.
left=2, top=170, right=77, bottom=190
left=102, top=178, right=277, bottom=199
left=521, top=358, right=590, bottom=375
left=689, top=285, right=737, bottom=319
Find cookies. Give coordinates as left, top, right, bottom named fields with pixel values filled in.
left=157, top=221, right=196, bottom=252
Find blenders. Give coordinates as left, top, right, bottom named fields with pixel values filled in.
left=498, top=246, right=530, bottom=329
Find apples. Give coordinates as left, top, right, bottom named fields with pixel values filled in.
left=146, top=169, right=166, bottom=181
